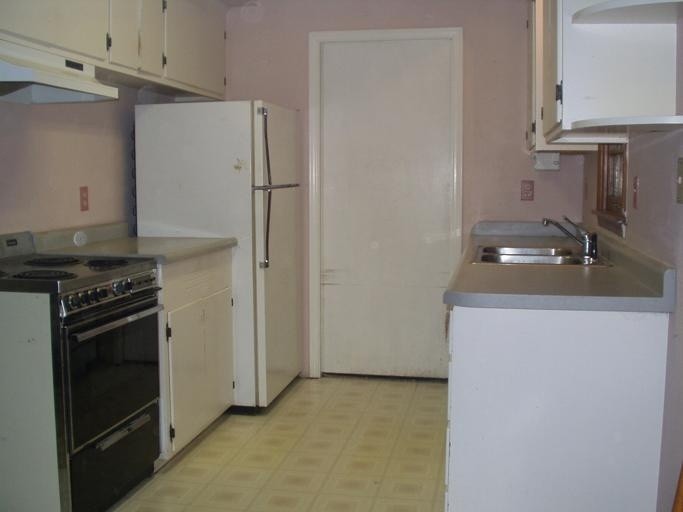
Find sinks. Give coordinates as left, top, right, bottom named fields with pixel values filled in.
left=468, top=253, right=615, bottom=268
left=477, top=243, right=588, bottom=255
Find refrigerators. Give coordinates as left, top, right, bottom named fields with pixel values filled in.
left=134, top=98, right=302, bottom=411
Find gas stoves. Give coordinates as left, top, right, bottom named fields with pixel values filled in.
left=0, top=230, right=158, bottom=292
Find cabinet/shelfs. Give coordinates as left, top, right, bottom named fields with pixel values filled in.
left=1, top=0, right=228, bottom=97
left=525, top=0, right=683, bottom=154
left=157, top=249, right=235, bottom=460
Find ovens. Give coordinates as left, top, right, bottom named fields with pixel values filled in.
left=58, top=294, right=164, bottom=512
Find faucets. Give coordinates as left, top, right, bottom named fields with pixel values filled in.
left=541, top=213, right=599, bottom=260
